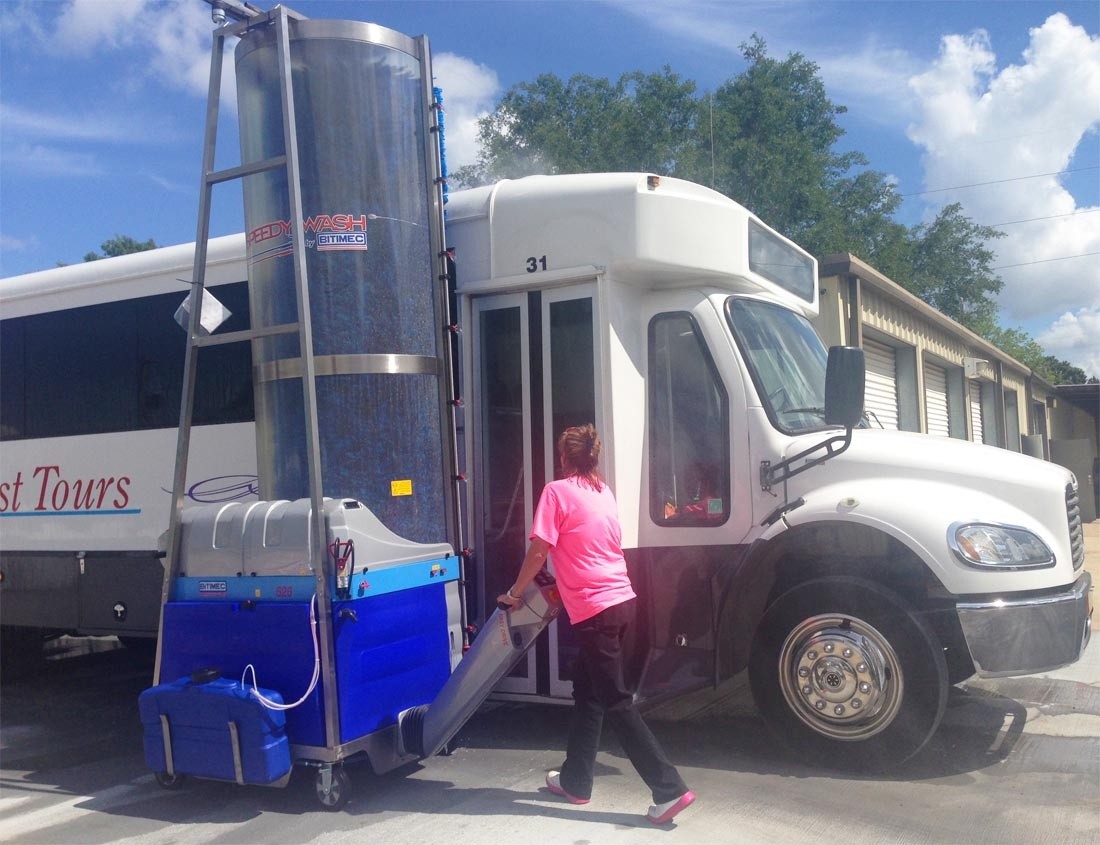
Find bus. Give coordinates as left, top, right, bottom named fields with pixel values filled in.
left=0, top=172, right=1097, bottom=781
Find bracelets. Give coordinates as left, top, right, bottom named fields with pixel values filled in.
left=506, top=587, right=521, bottom=601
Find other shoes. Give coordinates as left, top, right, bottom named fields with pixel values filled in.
left=546, top=771, right=590, bottom=804
left=648, top=791, right=696, bottom=825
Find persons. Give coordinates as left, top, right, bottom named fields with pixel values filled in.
left=665, top=462, right=722, bottom=520
left=496, top=424, right=697, bottom=824
